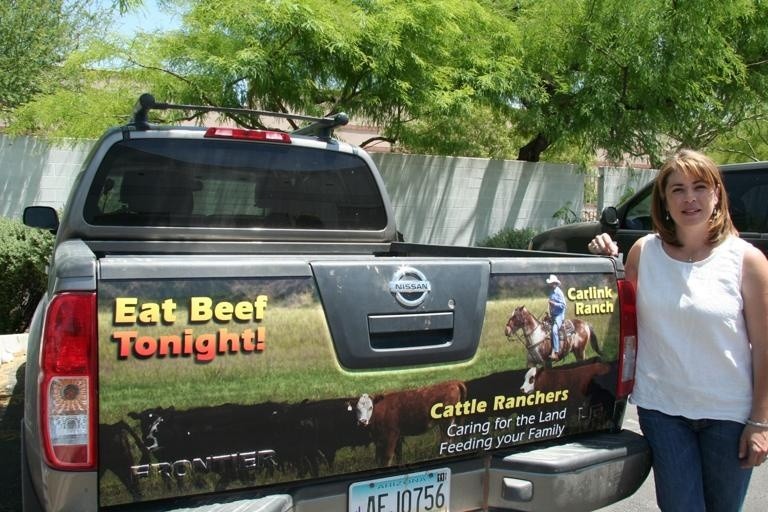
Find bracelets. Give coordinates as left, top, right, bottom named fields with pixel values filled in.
left=746, top=418, right=768, bottom=430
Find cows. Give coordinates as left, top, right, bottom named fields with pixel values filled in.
left=97, top=356, right=622, bottom=504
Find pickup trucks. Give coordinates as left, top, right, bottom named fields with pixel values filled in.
left=17, top=95, right=650, bottom=510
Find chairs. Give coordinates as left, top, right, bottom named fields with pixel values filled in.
left=103, top=170, right=326, bottom=227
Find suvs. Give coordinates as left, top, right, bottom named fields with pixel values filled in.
left=525, top=162, right=768, bottom=265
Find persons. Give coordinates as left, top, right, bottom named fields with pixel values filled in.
left=587, top=148, right=768, bottom=511
left=544, top=273, right=566, bottom=360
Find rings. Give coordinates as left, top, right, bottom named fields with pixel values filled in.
left=592, top=243, right=597, bottom=248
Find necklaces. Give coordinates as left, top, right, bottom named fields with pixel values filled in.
left=676, top=243, right=706, bottom=262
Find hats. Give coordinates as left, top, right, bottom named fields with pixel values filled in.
left=546, top=275, right=559, bottom=285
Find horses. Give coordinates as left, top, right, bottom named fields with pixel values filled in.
left=505, top=304, right=603, bottom=368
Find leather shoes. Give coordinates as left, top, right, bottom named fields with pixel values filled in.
left=550, top=352, right=559, bottom=359
